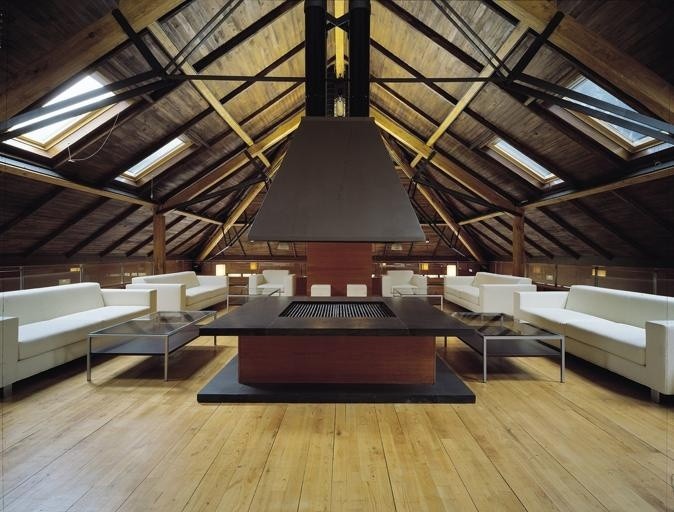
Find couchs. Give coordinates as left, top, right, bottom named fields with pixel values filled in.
left=381, top=270, right=427, bottom=298
left=0, top=281, right=157, bottom=403
left=310, top=284, right=332, bottom=297
left=443, top=272, right=537, bottom=323
left=348, top=284, right=367, bottom=297
left=126, top=271, right=229, bottom=311
left=513, top=284, right=674, bottom=402
left=248, top=270, right=297, bottom=296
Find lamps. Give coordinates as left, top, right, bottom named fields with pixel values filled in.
left=334, top=95, right=346, bottom=118
